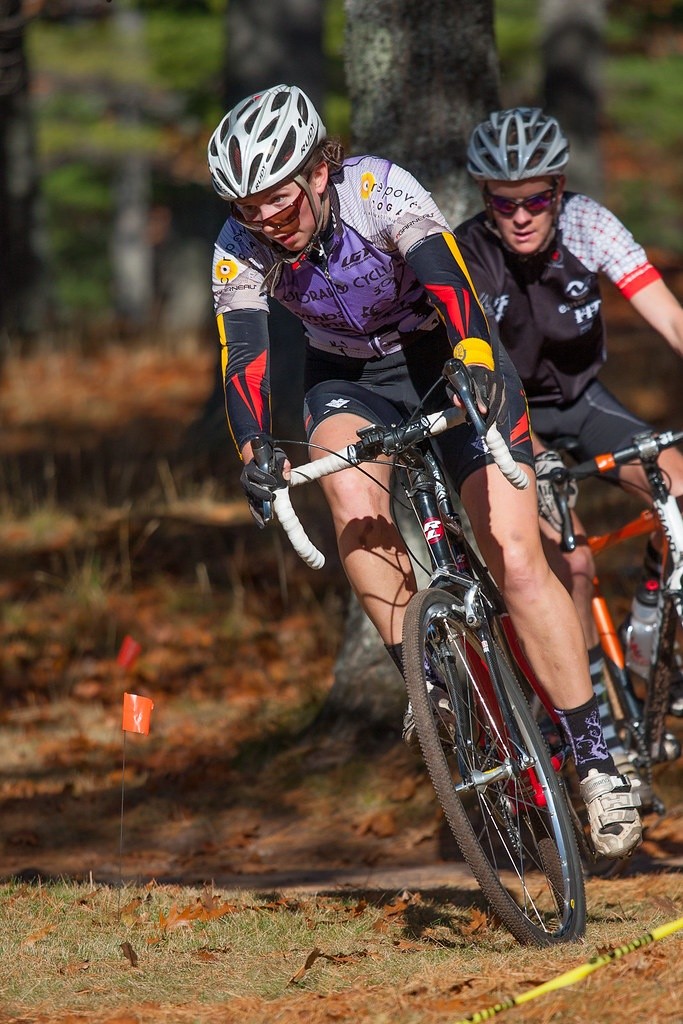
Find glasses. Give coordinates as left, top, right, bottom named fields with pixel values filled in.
left=482, top=177, right=559, bottom=214
left=230, top=170, right=312, bottom=232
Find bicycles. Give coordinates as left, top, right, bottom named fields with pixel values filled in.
left=252, top=359, right=590, bottom=954
left=536, top=427, right=683, bottom=864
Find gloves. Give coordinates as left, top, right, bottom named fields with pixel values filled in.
left=534, top=450, right=578, bottom=533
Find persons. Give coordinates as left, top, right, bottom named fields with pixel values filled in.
left=208, top=81, right=644, bottom=856
left=449, top=106, right=683, bottom=805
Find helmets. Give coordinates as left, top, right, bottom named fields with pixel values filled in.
left=467, top=107, right=571, bottom=180
left=206, top=84, right=325, bottom=201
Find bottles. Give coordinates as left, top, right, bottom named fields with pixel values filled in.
left=627, top=579, right=660, bottom=665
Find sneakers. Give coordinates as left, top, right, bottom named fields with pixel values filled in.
left=578, top=767, right=643, bottom=859
left=612, top=750, right=654, bottom=806
left=402, top=681, right=456, bottom=759
